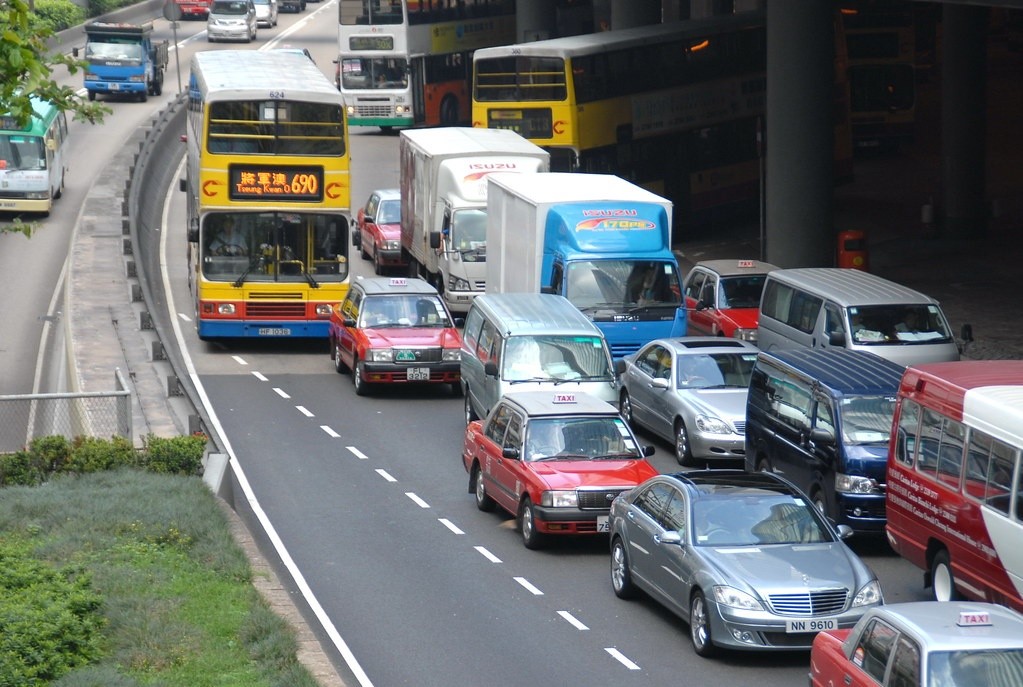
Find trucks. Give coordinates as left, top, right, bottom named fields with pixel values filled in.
left=400, top=127, right=551, bottom=322
left=72, top=20, right=169, bottom=102
left=487, top=172, right=688, bottom=378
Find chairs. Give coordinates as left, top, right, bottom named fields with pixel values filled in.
left=757, top=506, right=792, bottom=543
left=208, top=124, right=260, bottom=154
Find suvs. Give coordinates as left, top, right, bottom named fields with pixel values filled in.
left=328, top=274, right=464, bottom=396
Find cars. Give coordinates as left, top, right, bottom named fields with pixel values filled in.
left=609, top=470, right=885, bottom=658
left=617, top=336, right=758, bottom=467
left=682, top=260, right=782, bottom=347
left=358, top=189, right=401, bottom=274
left=808, top=601, right=1023, bottom=687
left=173, top=0, right=320, bottom=42
left=462, top=390, right=661, bottom=549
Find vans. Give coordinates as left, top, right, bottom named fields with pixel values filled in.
left=745, top=349, right=907, bottom=547
left=460, top=292, right=621, bottom=425
left=759, top=268, right=974, bottom=368
left=885, top=361, right=1023, bottom=614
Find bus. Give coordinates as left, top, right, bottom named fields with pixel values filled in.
left=334, top=0, right=516, bottom=134
left=471, top=10, right=769, bottom=241
left=180, top=49, right=363, bottom=341
left=0, top=94, right=69, bottom=218
left=830, top=9, right=933, bottom=157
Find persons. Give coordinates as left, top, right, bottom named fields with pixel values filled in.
left=835, top=309, right=865, bottom=335
left=631, top=265, right=676, bottom=303
left=209, top=218, right=248, bottom=257
left=892, top=309, right=921, bottom=334
left=525, top=428, right=565, bottom=461
left=669, top=357, right=709, bottom=386
